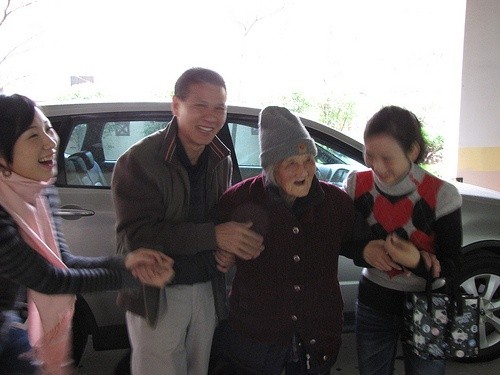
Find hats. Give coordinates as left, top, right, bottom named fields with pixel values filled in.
left=259, top=106, right=318, bottom=167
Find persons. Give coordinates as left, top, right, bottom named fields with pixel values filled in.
left=215, top=106, right=440, bottom=374
left=341, top=105, right=464, bottom=375
left=0, top=93, right=175, bottom=375
left=112, top=67, right=265, bottom=375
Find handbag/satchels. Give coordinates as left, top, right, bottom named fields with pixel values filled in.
left=402, top=258, right=481, bottom=360
left=207, top=323, right=310, bottom=375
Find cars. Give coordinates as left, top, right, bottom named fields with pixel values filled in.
left=24, top=103, right=500, bottom=368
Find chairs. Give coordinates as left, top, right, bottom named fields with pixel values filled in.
left=65, top=151, right=108, bottom=187
